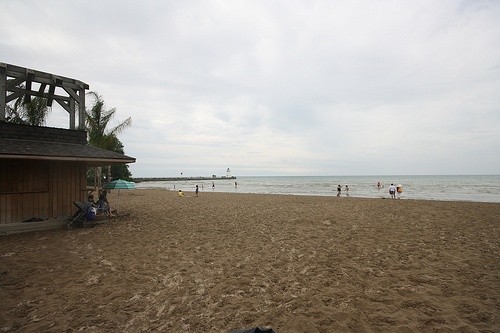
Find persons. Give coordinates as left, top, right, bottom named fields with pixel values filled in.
left=344, top=185, right=349, bottom=195
left=88, top=195, right=96, bottom=208
left=337, top=184, right=342, bottom=197
left=389, top=183, right=396, bottom=198
left=178, top=190, right=185, bottom=197
left=195, top=185, right=199, bottom=196
left=212, top=182, right=215, bottom=187
left=99, top=190, right=111, bottom=214
left=396, top=184, right=402, bottom=199
left=377, top=182, right=381, bottom=188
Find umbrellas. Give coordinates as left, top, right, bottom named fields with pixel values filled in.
left=102, top=178, right=136, bottom=214
